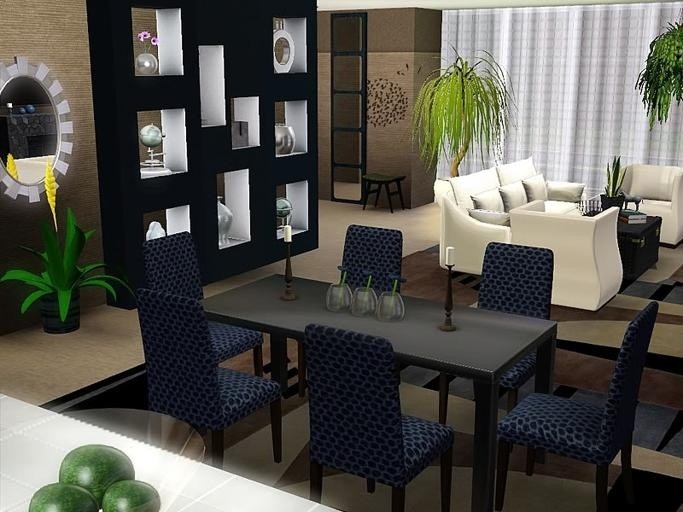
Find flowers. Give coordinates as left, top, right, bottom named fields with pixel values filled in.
left=1, top=157, right=136, bottom=318
left=5, top=152, right=21, bottom=180
left=135, top=30, right=158, bottom=53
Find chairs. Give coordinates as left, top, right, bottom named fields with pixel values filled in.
left=136, top=293, right=286, bottom=471
left=437, top=239, right=553, bottom=426
left=325, top=222, right=402, bottom=299
left=305, top=321, right=456, bottom=511
left=495, top=296, right=658, bottom=509
left=134, top=229, right=268, bottom=379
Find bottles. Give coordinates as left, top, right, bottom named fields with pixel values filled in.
left=375, top=291, right=405, bottom=322
left=350, top=286, right=377, bottom=317
left=325, top=283, right=351, bottom=312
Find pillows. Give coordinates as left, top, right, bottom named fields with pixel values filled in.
left=521, top=172, right=548, bottom=202
left=499, top=179, right=527, bottom=212
left=544, top=178, right=585, bottom=202
left=469, top=186, right=505, bottom=212
left=467, top=206, right=510, bottom=228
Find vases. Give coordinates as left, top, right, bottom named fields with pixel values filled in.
left=217, top=196, right=234, bottom=247
left=135, top=54, right=159, bottom=75
left=39, top=289, right=82, bottom=333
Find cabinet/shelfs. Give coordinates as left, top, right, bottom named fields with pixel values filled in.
left=83, top=2, right=319, bottom=314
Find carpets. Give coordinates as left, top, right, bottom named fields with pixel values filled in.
left=31, top=236, right=681, bottom=512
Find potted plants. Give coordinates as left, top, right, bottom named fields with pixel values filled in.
left=600, top=155, right=627, bottom=209
left=407, top=40, right=519, bottom=208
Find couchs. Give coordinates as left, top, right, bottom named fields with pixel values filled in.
left=612, top=161, right=682, bottom=247
left=433, top=154, right=586, bottom=277
left=511, top=205, right=625, bottom=315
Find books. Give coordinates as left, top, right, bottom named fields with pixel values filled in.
left=618, top=215, right=647, bottom=225
left=619, top=207, right=647, bottom=219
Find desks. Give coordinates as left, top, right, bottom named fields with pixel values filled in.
left=198, top=274, right=558, bottom=512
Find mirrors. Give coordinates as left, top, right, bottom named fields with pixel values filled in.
left=272, top=30, right=295, bottom=73
left=0, top=54, right=76, bottom=201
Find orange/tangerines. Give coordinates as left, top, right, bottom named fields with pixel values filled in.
left=28, top=484, right=97, bottom=512
left=60, top=444, right=136, bottom=509
left=102, top=480, right=160, bottom=512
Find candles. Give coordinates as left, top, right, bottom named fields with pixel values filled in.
left=445, top=246, right=454, bottom=266
left=282, top=224, right=291, bottom=241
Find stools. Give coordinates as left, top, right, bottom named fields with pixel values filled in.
left=67, top=407, right=208, bottom=462
left=359, top=172, right=408, bottom=212
